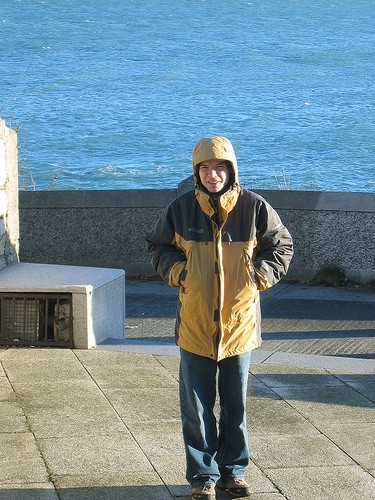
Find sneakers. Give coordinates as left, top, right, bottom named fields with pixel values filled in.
left=192, top=486, right=216, bottom=500
left=216, top=478, right=251, bottom=497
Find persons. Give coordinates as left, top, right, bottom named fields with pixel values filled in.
left=143, top=135, right=296, bottom=500
